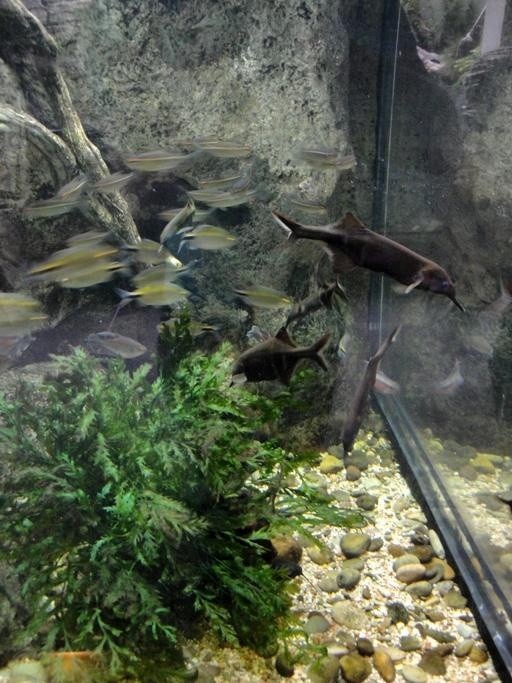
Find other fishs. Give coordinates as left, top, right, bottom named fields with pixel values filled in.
left=0, top=139, right=467, bottom=389
